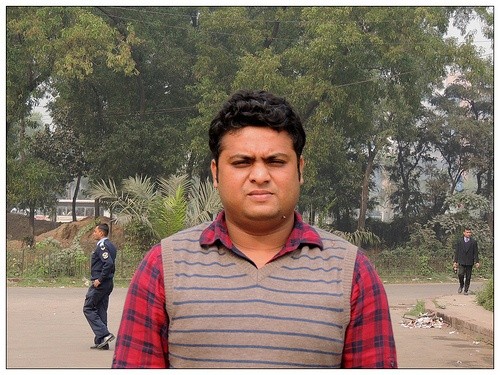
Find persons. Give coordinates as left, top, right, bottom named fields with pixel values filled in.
left=83, top=223, right=116, bottom=350
left=110, top=88, right=398, bottom=369
left=453, top=228, right=479, bottom=295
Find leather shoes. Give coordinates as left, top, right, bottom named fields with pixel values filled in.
left=90, top=342, right=109, bottom=350
left=97, top=333, right=115, bottom=349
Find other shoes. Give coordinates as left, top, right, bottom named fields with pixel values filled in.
left=464, top=290, right=468, bottom=295
left=458, top=287, right=463, bottom=293
